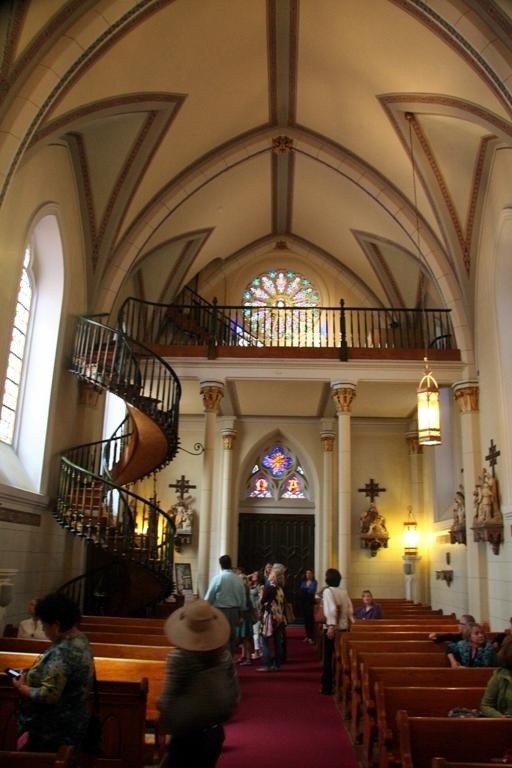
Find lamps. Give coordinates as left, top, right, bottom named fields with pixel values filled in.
left=404, top=108, right=443, bottom=447
left=402, top=505, right=420, bottom=557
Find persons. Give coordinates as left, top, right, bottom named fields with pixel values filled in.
left=318, top=568, right=355, bottom=695
left=351, top=589, right=381, bottom=619
left=451, top=484, right=466, bottom=526
left=300, top=566, right=318, bottom=644
left=358, top=503, right=387, bottom=533
left=479, top=635, right=511, bottom=718
left=429, top=610, right=511, bottom=667
left=202, top=553, right=288, bottom=674
left=166, top=502, right=193, bottom=528
left=11, top=594, right=99, bottom=752
left=155, top=601, right=245, bottom=767
left=473, top=468, right=502, bottom=522
left=17, top=598, right=53, bottom=639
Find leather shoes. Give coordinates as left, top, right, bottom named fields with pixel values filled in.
left=236, top=651, right=280, bottom=672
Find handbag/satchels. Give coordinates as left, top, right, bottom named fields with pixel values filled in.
left=312, top=597, right=341, bottom=626
left=250, top=608, right=261, bottom=625
left=284, top=601, right=297, bottom=625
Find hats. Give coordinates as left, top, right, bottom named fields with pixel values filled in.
left=162, top=598, right=232, bottom=653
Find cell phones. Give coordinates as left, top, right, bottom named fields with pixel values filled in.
left=4, top=667, right=21, bottom=680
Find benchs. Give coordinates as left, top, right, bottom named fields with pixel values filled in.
left=298, top=590, right=512, bottom=767
left=0, top=609, right=183, bottom=767
